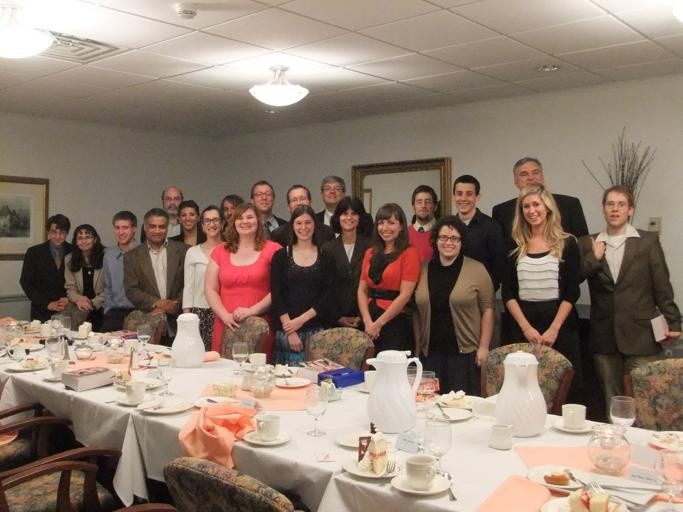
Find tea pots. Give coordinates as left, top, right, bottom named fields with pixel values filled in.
left=495, top=349, right=548, bottom=438
left=170, top=313, right=206, bottom=369
left=365, top=350, right=423, bottom=436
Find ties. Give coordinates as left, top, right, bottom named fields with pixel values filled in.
left=54, top=247, right=62, bottom=269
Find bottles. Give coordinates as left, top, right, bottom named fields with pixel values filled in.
left=128, top=347, right=136, bottom=377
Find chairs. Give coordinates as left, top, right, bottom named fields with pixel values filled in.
left=163, top=457, right=295, bottom=512
left=54, top=297, right=90, bottom=333
left=0, top=461, right=178, bottom=512
left=303, top=326, right=375, bottom=371
left=624, top=358, right=683, bottom=430
left=0, top=424, right=121, bottom=512
left=123, top=309, right=167, bottom=344
left=0, top=400, right=73, bottom=467
left=481, top=344, right=574, bottom=418
left=221, top=316, right=269, bottom=362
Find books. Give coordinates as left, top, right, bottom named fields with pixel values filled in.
left=63, top=363, right=118, bottom=392
left=650, top=314, right=671, bottom=343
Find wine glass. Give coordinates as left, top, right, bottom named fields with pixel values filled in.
left=415, top=370, right=436, bottom=405
left=304, top=389, right=331, bottom=439
left=46, top=340, right=67, bottom=360
left=62, top=317, right=72, bottom=332
left=651, top=449, right=682, bottom=512
left=231, top=342, right=248, bottom=376
left=137, top=325, right=151, bottom=349
left=52, top=315, right=63, bottom=340
left=610, top=395, right=636, bottom=441
left=424, top=417, right=453, bottom=473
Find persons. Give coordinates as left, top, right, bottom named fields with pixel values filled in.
left=100, top=211, right=142, bottom=333
left=411, top=215, right=495, bottom=399
left=354, top=202, right=424, bottom=371
left=269, top=185, right=338, bottom=248
left=503, top=182, right=583, bottom=416
left=270, top=204, right=339, bottom=366
left=250, top=180, right=288, bottom=236
left=63, top=224, right=107, bottom=334
left=452, top=174, right=504, bottom=294
left=168, top=199, right=209, bottom=247
left=576, top=183, right=683, bottom=422
left=162, top=185, right=182, bottom=242
left=202, top=202, right=284, bottom=367
left=181, top=205, right=225, bottom=352
left=314, top=175, right=376, bottom=242
left=122, top=207, right=193, bottom=348
left=491, top=156, right=598, bottom=291
left=219, top=194, right=246, bottom=243
left=17, top=213, right=73, bottom=325
left=406, top=185, right=440, bottom=263
left=322, top=195, right=379, bottom=334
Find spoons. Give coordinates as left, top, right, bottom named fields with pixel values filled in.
left=206, top=398, right=239, bottom=404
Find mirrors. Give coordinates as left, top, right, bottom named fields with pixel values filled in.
left=362, top=188, right=372, bottom=214
left=351, top=157, right=452, bottom=227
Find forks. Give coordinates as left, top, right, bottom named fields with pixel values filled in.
left=591, top=481, right=642, bottom=509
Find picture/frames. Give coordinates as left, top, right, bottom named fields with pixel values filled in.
left=0, top=175, right=50, bottom=261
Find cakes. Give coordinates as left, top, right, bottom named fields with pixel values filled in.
left=568, top=491, right=672, bottom=512
left=439, top=389, right=465, bottom=407
left=358, top=422, right=396, bottom=476
left=544, top=473, right=570, bottom=485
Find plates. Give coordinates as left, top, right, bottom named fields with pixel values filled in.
left=194, top=396, right=239, bottom=409
left=243, top=430, right=292, bottom=447
left=8, top=361, right=47, bottom=374
left=275, top=377, right=313, bottom=389
left=392, top=471, right=452, bottom=497
left=8, top=342, right=43, bottom=351
left=114, top=372, right=165, bottom=392
left=118, top=397, right=146, bottom=406
left=42, top=373, right=62, bottom=381
left=434, top=408, right=474, bottom=423
left=527, top=465, right=590, bottom=488
left=139, top=396, right=195, bottom=414
left=539, top=495, right=632, bottom=512
left=342, top=456, right=402, bottom=479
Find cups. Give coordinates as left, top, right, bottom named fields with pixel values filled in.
left=84, top=322, right=92, bottom=334
left=406, top=454, right=440, bottom=494
left=51, top=362, right=68, bottom=377
left=491, top=424, right=515, bottom=451
left=249, top=352, right=267, bottom=368
left=363, top=370, right=381, bottom=390
left=561, top=404, right=586, bottom=432
left=257, top=416, right=281, bottom=439
left=78, top=325, right=89, bottom=338
left=125, top=380, right=147, bottom=402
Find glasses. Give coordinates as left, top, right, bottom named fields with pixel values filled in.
left=74, top=236, right=99, bottom=241
left=323, top=187, right=344, bottom=194
left=253, top=191, right=275, bottom=200
left=162, top=195, right=183, bottom=203
left=437, top=235, right=463, bottom=242
left=603, top=200, right=631, bottom=210
left=202, top=218, right=223, bottom=226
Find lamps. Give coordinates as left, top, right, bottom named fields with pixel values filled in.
left=249, top=67, right=309, bottom=107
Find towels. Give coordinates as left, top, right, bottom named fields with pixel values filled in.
left=474, top=475, right=551, bottom=511
left=178, top=402, right=257, bottom=469
